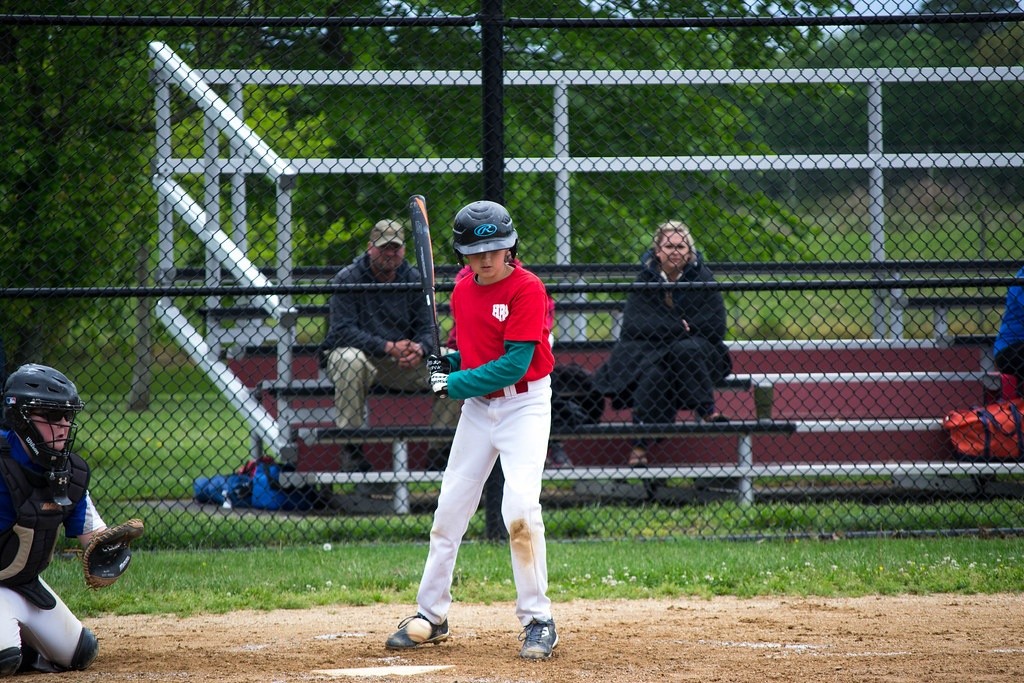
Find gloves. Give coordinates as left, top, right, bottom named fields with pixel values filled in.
left=427, top=355, right=451, bottom=374
left=428, top=372, right=449, bottom=400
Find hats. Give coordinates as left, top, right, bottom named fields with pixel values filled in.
left=370, top=220, right=404, bottom=248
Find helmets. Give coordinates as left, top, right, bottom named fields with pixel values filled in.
left=3, top=363, right=84, bottom=471
left=451, top=201, right=519, bottom=270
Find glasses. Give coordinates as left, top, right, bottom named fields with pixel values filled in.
left=27, top=409, right=73, bottom=422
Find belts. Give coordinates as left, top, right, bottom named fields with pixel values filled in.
left=484, top=382, right=529, bottom=400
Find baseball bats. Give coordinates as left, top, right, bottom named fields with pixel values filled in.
left=406, top=194, right=449, bottom=399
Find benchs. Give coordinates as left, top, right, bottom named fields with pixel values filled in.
left=169, top=256, right=1024, bottom=483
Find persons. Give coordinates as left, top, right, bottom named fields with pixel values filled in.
left=606, top=222, right=733, bottom=467
left=318, top=218, right=460, bottom=471
left=994, top=266, right=1024, bottom=403
left=384, top=203, right=562, bottom=663
left=0, top=362, right=143, bottom=676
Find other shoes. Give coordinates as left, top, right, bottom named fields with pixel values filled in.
left=338, top=449, right=372, bottom=472
left=709, top=413, right=728, bottom=423
left=629, top=450, right=647, bottom=467
left=427, top=451, right=448, bottom=472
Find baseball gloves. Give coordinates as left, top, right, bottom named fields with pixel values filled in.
left=82, top=519, right=145, bottom=587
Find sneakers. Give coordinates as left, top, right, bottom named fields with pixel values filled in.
left=519, top=618, right=558, bottom=660
left=385, top=612, right=449, bottom=651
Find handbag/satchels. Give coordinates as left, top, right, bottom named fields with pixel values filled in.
left=190, top=455, right=312, bottom=506
left=551, top=365, right=607, bottom=434
left=945, top=398, right=1024, bottom=459
